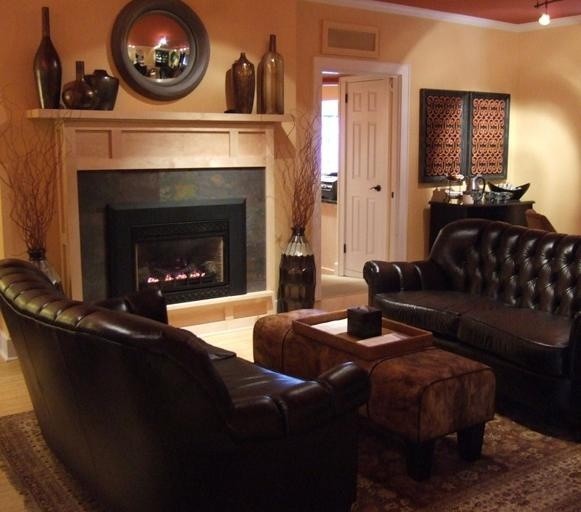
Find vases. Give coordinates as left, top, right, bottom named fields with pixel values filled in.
left=26, top=248, right=64, bottom=293
left=278, top=226, right=317, bottom=315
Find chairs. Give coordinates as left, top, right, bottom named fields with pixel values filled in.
left=526, top=209, right=558, bottom=233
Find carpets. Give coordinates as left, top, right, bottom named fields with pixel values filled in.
left=0, top=410, right=581, bottom=511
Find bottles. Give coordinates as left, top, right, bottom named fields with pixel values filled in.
left=32, top=6, right=62, bottom=111
left=229, top=32, right=283, bottom=113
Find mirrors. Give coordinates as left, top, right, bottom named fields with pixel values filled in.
left=111, top=1, right=211, bottom=102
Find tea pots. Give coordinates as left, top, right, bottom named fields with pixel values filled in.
left=466, top=174, right=486, bottom=201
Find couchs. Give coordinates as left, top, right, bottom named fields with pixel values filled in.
left=363, top=217, right=581, bottom=445
left=0, top=256, right=373, bottom=511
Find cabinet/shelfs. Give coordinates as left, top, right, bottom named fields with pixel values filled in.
left=428, top=200, right=536, bottom=254
